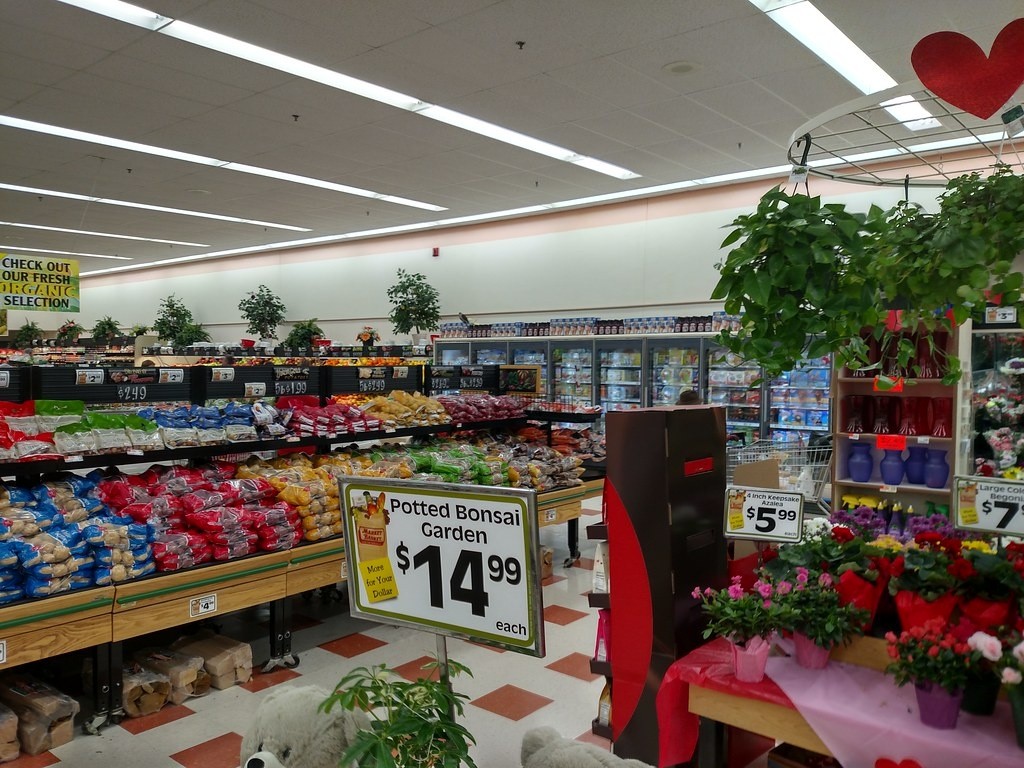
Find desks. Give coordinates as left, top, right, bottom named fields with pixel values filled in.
left=653, top=633, right=1024, bottom=768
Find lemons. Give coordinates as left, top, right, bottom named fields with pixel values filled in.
left=408, top=360, right=425, bottom=365
left=337, top=358, right=366, bottom=366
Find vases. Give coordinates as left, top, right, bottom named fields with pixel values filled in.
left=894, top=591, right=956, bottom=631
left=914, top=680, right=963, bottom=728
left=363, top=337, right=373, bottom=346
left=957, top=593, right=1015, bottom=629
left=793, top=628, right=833, bottom=670
left=732, top=643, right=767, bottom=683
left=880, top=444, right=906, bottom=484
left=960, top=671, right=1001, bottom=715
left=67, top=333, right=79, bottom=339
left=836, top=574, right=885, bottom=633
left=904, top=447, right=928, bottom=483
left=848, top=443, right=873, bottom=481
left=924, top=450, right=949, bottom=487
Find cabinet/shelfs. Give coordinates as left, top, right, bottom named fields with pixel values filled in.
left=434, top=318, right=1024, bottom=536
left=1, top=337, right=605, bottom=767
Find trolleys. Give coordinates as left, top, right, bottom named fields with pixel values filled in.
left=725, top=438, right=833, bottom=517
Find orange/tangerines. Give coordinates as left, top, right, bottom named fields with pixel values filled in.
left=366, top=357, right=405, bottom=366
left=332, top=394, right=375, bottom=406
left=324, top=358, right=336, bottom=365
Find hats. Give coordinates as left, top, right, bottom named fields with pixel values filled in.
left=676, top=390, right=703, bottom=405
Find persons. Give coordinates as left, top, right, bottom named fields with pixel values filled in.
left=675, top=390, right=740, bottom=443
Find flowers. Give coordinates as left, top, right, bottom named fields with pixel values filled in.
left=356, top=327, right=380, bottom=342
left=57, top=319, right=86, bottom=339
left=691, top=507, right=1024, bottom=685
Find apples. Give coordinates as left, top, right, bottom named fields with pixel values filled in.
left=200, top=358, right=323, bottom=365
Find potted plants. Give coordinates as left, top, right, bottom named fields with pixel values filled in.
left=386, top=266, right=442, bottom=345
left=88, top=315, right=127, bottom=344
left=151, top=293, right=212, bottom=350
left=129, top=325, right=149, bottom=337
left=14, top=316, right=45, bottom=344
left=237, top=284, right=287, bottom=341
left=282, top=319, right=325, bottom=347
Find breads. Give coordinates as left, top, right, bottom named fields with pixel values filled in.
left=0, top=442, right=506, bottom=609
